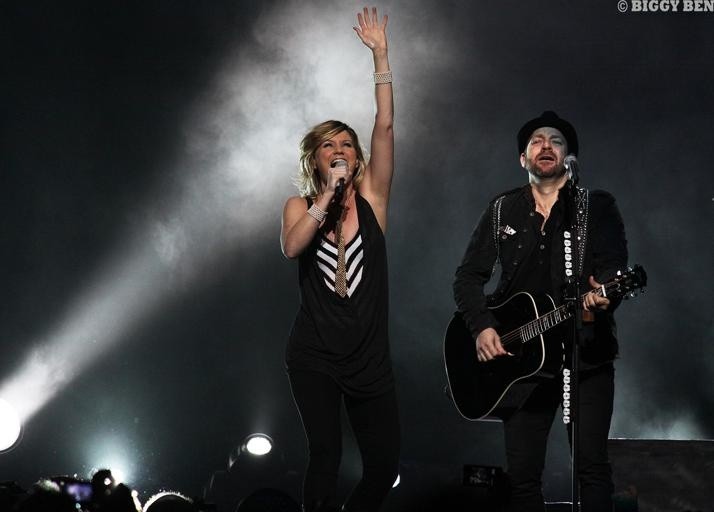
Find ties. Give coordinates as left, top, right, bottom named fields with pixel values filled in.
left=334, top=204, right=347, bottom=298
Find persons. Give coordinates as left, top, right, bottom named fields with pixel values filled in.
left=280, top=7, right=400, bottom=512
left=453, top=111, right=627, bottom=512
left=3, top=459, right=636, bottom=512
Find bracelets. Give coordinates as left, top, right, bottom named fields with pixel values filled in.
left=373, top=72, right=393, bottom=84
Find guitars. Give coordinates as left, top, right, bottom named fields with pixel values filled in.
left=443, top=263, right=647, bottom=422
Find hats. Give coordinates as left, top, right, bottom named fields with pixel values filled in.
left=517, top=110, right=579, bottom=157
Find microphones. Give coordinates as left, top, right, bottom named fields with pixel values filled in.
left=335, top=158, right=348, bottom=201
left=564, top=154, right=580, bottom=189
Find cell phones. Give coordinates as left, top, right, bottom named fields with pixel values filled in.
left=463, top=464, right=504, bottom=487
left=64, top=480, right=94, bottom=504
